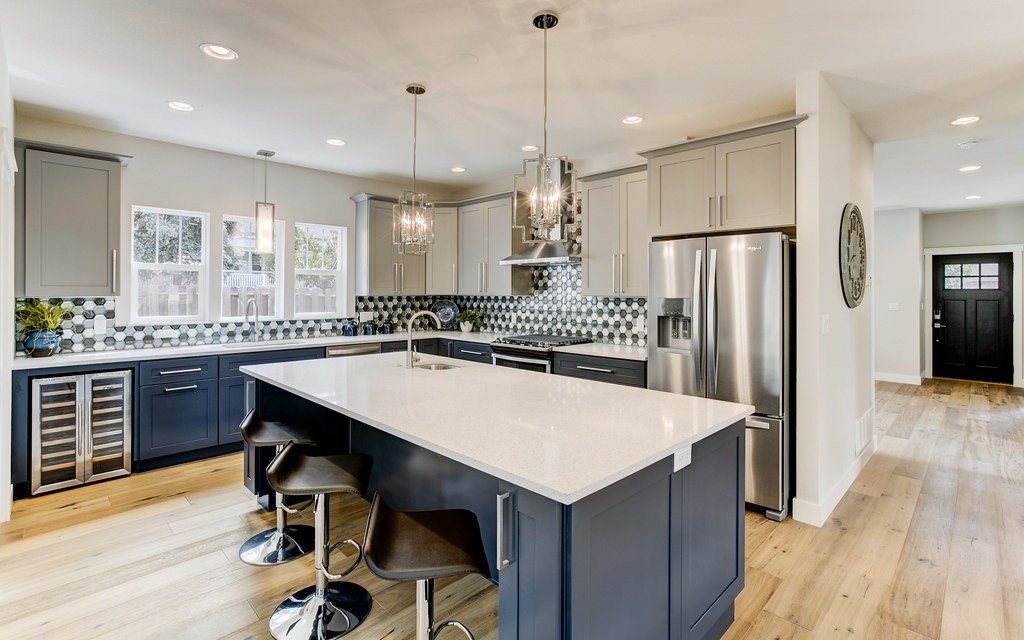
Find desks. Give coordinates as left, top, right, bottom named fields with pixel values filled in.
left=239, top=349, right=756, bottom=640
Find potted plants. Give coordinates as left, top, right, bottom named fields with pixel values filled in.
left=14, top=297, right=74, bottom=356
left=455, top=309, right=484, bottom=332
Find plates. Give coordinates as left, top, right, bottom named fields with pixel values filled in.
left=429, top=299, right=460, bottom=329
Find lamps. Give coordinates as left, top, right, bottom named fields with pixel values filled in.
left=255, top=149, right=276, bottom=255
left=512, top=9, right=579, bottom=243
left=392, top=82, right=436, bottom=256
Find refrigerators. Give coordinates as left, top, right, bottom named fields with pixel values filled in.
left=645, top=230, right=796, bottom=522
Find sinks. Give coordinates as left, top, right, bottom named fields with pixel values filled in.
left=415, top=363, right=463, bottom=370
left=221, top=339, right=309, bottom=349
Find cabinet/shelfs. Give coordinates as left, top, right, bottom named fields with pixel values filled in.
left=132, top=348, right=326, bottom=472
left=554, top=351, right=647, bottom=387
left=576, top=163, right=648, bottom=299
left=636, top=114, right=808, bottom=237
left=30, top=369, right=131, bottom=496
left=382, top=339, right=493, bottom=363
left=15, top=138, right=134, bottom=298
left=349, top=191, right=534, bottom=296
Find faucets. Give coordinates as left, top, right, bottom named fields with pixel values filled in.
left=242, top=299, right=260, bottom=341
left=406, top=310, right=442, bottom=369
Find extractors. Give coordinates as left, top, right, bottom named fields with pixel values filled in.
left=498, top=158, right=581, bottom=267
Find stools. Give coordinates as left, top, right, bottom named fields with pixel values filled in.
left=240, top=407, right=492, bottom=640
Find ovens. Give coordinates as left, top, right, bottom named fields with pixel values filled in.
left=491, top=352, right=554, bottom=374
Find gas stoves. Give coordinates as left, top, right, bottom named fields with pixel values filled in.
left=490, top=334, right=593, bottom=361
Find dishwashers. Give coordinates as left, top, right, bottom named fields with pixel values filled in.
left=326, top=342, right=382, bottom=358
